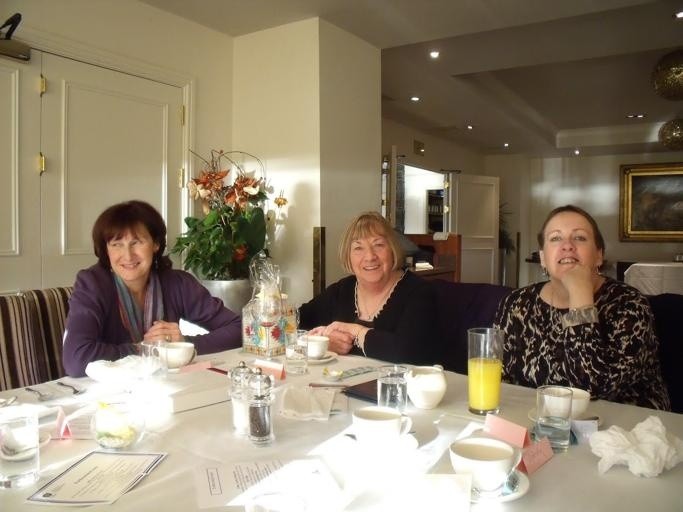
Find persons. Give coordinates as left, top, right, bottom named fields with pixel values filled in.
left=61, top=200, right=243, bottom=377
left=298, top=209, right=457, bottom=364
left=492, top=205, right=671, bottom=412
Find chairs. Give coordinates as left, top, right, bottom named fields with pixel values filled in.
left=0, top=286, right=73, bottom=392
left=642, top=294, right=683, bottom=414
left=423, top=279, right=509, bottom=374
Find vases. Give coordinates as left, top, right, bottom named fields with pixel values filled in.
left=202, top=281, right=252, bottom=316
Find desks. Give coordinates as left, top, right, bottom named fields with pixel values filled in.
left=624, top=262, right=683, bottom=298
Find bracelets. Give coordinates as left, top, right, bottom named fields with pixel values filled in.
left=569, top=303, right=595, bottom=310
left=352, top=322, right=367, bottom=345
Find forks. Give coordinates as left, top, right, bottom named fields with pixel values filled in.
left=57, top=381, right=87, bottom=396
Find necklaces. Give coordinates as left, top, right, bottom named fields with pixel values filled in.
left=358, top=276, right=398, bottom=319
left=547, top=278, right=599, bottom=336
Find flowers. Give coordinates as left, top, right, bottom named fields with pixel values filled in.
left=167, top=149, right=288, bottom=280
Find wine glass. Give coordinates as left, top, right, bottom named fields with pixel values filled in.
left=250, top=283, right=281, bottom=364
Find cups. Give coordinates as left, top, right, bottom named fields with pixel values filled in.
left=544, top=388, right=590, bottom=419
left=282, top=331, right=309, bottom=375
left=467, top=327, right=504, bottom=415
left=377, top=365, right=410, bottom=416
left=0, top=405, right=40, bottom=491
left=152, top=342, right=197, bottom=366
left=449, top=438, right=522, bottom=491
left=536, top=386, right=572, bottom=453
left=297, top=335, right=329, bottom=357
left=351, top=407, right=410, bottom=436
left=142, top=341, right=169, bottom=380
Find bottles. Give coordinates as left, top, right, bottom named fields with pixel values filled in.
left=245, top=367, right=277, bottom=446
left=228, top=359, right=252, bottom=438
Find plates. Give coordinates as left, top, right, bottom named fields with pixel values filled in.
left=528, top=405, right=601, bottom=430
left=307, top=351, right=337, bottom=363
left=40, top=429, right=52, bottom=456
left=451, top=469, right=530, bottom=502
left=168, top=368, right=181, bottom=374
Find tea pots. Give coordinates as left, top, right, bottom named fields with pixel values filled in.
left=404, top=365, right=447, bottom=409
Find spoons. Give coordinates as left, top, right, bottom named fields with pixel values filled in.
left=24, top=387, right=54, bottom=402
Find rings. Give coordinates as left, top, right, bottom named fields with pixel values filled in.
left=167, top=333, right=173, bottom=344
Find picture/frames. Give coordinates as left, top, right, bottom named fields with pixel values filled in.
left=618, top=162, right=683, bottom=242
